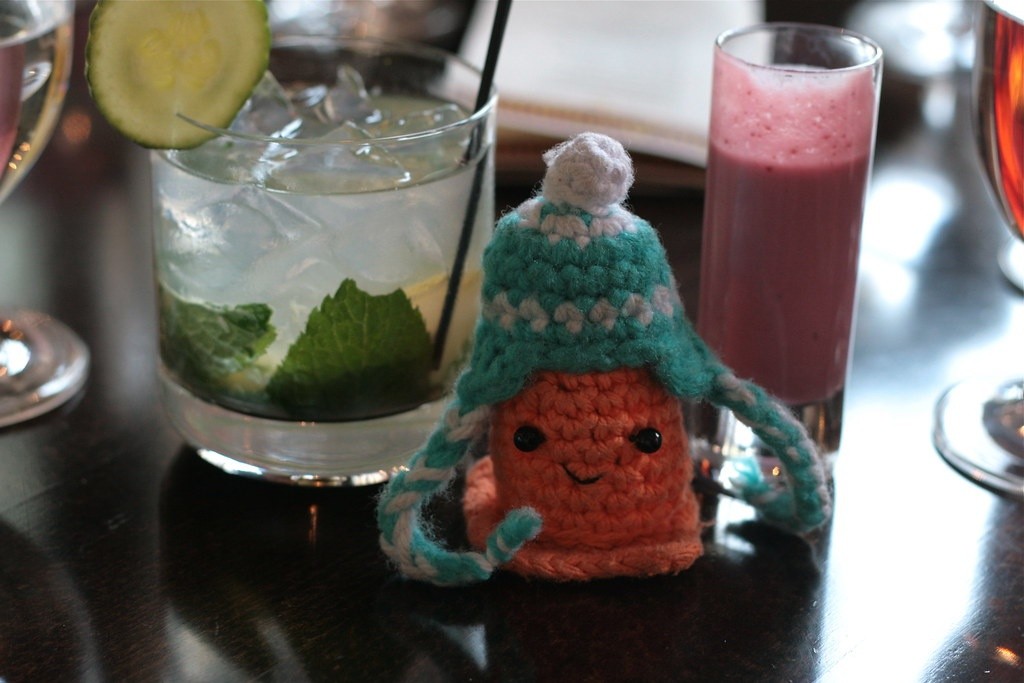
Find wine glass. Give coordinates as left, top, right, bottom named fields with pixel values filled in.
left=0, top=1, right=91, bottom=431
left=927, top=0, right=1024, bottom=495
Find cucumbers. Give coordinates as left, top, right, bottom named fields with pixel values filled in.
left=85, top=0, right=271, bottom=150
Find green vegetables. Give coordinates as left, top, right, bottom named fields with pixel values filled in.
left=155, top=278, right=433, bottom=420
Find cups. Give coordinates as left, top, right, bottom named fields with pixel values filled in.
left=696, top=18, right=884, bottom=461
left=151, top=39, right=501, bottom=492
